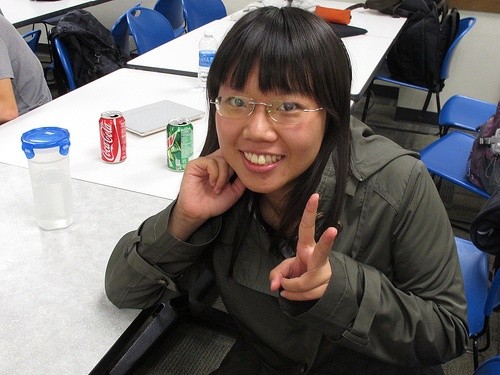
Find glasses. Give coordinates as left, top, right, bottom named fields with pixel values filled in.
left=209, top=95, right=325, bottom=124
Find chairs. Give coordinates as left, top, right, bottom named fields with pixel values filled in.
left=21, top=0, right=500, bottom=375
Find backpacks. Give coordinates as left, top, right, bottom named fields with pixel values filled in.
left=387, top=0, right=460, bottom=93
left=47, top=9, right=126, bottom=88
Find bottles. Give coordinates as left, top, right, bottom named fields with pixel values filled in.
left=197, top=33, right=219, bottom=100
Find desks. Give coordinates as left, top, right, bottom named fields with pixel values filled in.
left=0, top=159, right=242, bottom=375
left=126, top=19, right=394, bottom=103
left=0, top=65, right=356, bottom=200
left=0, top=0, right=115, bottom=30
left=219, top=0, right=408, bottom=41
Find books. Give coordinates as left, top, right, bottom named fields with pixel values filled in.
left=122, top=100, right=205, bottom=137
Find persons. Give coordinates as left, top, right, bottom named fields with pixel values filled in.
left=105, top=5, right=470, bottom=375
left=0, top=14, right=52, bottom=125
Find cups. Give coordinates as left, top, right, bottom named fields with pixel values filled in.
left=21, top=127, right=75, bottom=230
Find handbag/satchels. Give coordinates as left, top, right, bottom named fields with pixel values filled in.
left=467, top=101, right=500, bottom=195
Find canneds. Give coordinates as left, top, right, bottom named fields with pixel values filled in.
left=167, top=118, right=193, bottom=172
left=98, top=110, right=126, bottom=163
